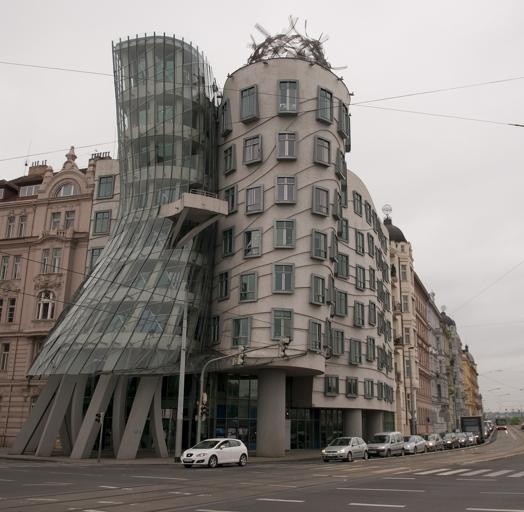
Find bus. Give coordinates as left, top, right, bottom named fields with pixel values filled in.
left=495, top=419, right=507, bottom=430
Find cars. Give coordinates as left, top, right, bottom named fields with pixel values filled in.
left=180, top=437, right=249, bottom=468
left=320, top=435, right=368, bottom=462
left=404, top=434, right=428, bottom=454
left=428, top=432, right=478, bottom=450
left=484, top=419, right=494, bottom=439
left=369, top=433, right=404, bottom=457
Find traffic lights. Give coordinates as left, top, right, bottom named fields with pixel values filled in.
left=94, top=411, right=105, bottom=425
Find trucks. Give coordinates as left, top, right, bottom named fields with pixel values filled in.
left=459, top=416, right=484, bottom=443
